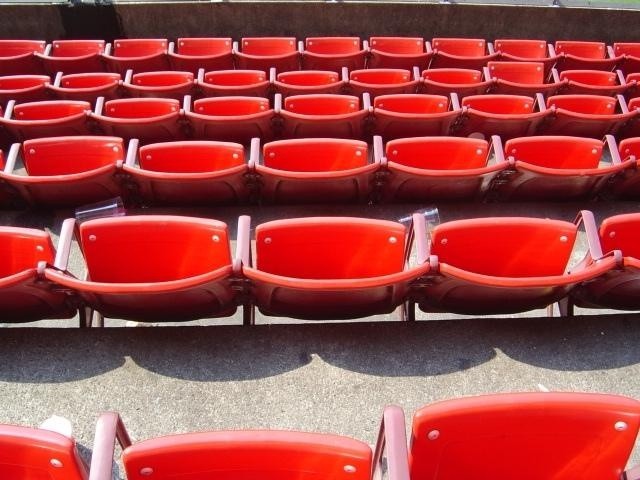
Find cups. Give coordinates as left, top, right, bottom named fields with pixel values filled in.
left=396, top=205, right=441, bottom=233
left=74, top=196, right=125, bottom=223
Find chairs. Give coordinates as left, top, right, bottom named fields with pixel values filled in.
left=404, top=205, right=622, bottom=319
left=88, top=407, right=411, bottom=479
left=370, top=392, right=640, bottom=479
left=1, top=397, right=127, bottom=480
left=0, top=225, right=92, bottom=328
left=235, top=213, right=427, bottom=320
left=39, top=215, right=255, bottom=329
left=568, top=209, right=640, bottom=317
